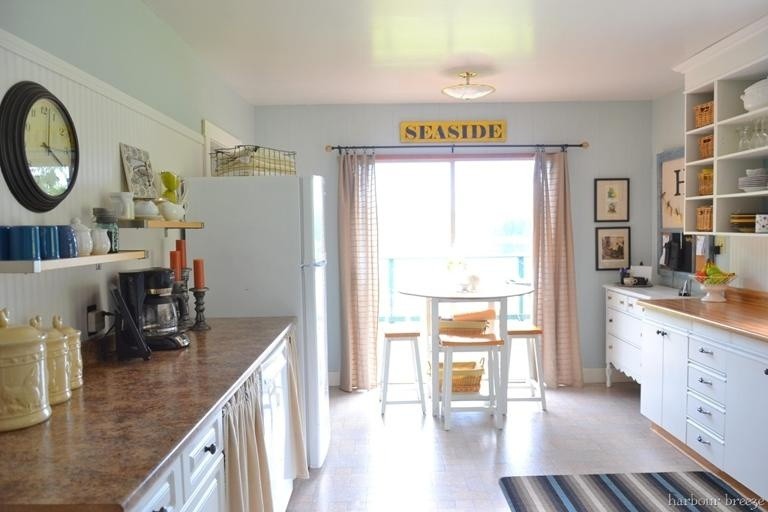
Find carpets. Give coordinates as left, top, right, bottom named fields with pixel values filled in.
left=493, top=464, right=765, bottom=511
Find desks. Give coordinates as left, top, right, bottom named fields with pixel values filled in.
left=389, top=272, right=539, bottom=433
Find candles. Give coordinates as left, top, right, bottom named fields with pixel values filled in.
left=175, top=238, right=188, bottom=270
left=193, top=258, right=205, bottom=290
left=170, top=250, right=182, bottom=282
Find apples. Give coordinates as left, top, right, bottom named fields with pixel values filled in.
left=695, top=271, right=706, bottom=283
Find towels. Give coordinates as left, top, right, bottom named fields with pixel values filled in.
left=215, top=143, right=299, bottom=178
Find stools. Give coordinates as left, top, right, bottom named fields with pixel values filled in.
left=380, top=327, right=427, bottom=418
left=496, top=318, right=549, bottom=414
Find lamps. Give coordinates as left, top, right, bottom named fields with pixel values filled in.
left=439, top=69, right=497, bottom=104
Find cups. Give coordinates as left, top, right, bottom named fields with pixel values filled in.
left=756, top=215, right=766, bottom=232
left=1, top=224, right=77, bottom=264
left=156, top=198, right=190, bottom=222
left=110, top=191, right=133, bottom=220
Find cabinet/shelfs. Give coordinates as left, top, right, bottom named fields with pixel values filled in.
left=724, top=324, right=768, bottom=505
left=120, top=327, right=303, bottom=512
left=638, top=296, right=691, bottom=448
left=602, top=279, right=702, bottom=389
left=681, top=55, right=767, bottom=234
left=685, top=315, right=737, bottom=480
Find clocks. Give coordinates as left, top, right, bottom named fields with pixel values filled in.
left=0, top=71, right=83, bottom=215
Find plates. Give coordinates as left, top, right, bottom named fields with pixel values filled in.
left=737, top=169, right=767, bottom=192
left=727, top=211, right=756, bottom=232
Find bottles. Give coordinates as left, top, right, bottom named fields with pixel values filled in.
left=620, top=261, right=648, bottom=285
left=69, top=206, right=120, bottom=255
left=28, top=313, right=84, bottom=407
left=135, top=200, right=159, bottom=219
left=1, top=307, right=53, bottom=433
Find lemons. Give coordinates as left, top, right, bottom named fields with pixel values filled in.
left=160, top=171, right=179, bottom=204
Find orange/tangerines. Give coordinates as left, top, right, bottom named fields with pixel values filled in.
left=707, top=268, right=719, bottom=276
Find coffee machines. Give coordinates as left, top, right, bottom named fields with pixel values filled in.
left=115, top=267, right=190, bottom=363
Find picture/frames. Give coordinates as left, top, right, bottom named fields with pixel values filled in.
left=593, top=225, right=632, bottom=272
left=592, top=175, right=632, bottom=223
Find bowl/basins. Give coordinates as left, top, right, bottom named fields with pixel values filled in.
left=739, top=78, right=768, bottom=111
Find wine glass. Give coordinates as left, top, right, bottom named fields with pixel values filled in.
left=733, top=117, right=768, bottom=151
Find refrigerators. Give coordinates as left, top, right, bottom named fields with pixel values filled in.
left=181, top=172, right=334, bottom=471
